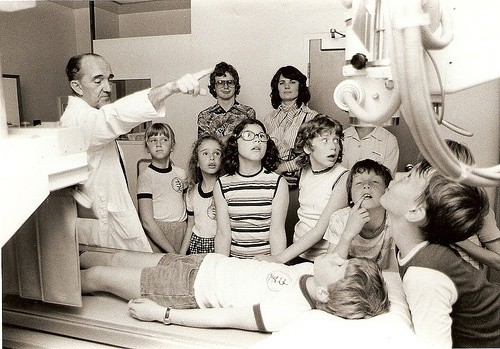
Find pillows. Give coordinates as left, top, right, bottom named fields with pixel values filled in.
left=276, top=270, right=415, bottom=349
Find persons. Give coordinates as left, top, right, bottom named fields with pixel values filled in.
left=253, top=113, right=351, bottom=267
left=59, top=52, right=215, bottom=252
left=418, top=139, right=500, bottom=279
left=212, top=117, right=290, bottom=260
left=378, top=139, right=500, bottom=348
left=341, top=122, right=401, bottom=178
left=79, top=252, right=392, bottom=332
left=136, top=122, right=188, bottom=254
left=179, top=136, right=227, bottom=255
left=323, top=159, right=393, bottom=270
left=261, top=65, right=320, bottom=248
left=197, top=62, right=256, bottom=146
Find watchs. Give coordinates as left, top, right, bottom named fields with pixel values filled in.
left=163, top=305, right=172, bottom=325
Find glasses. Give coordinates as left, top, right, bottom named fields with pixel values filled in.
left=214, top=80, right=237, bottom=88
left=236, top=131, right=269, bottom=142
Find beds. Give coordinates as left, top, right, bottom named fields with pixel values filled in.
left=2, top=244, right=272, bottom=349
